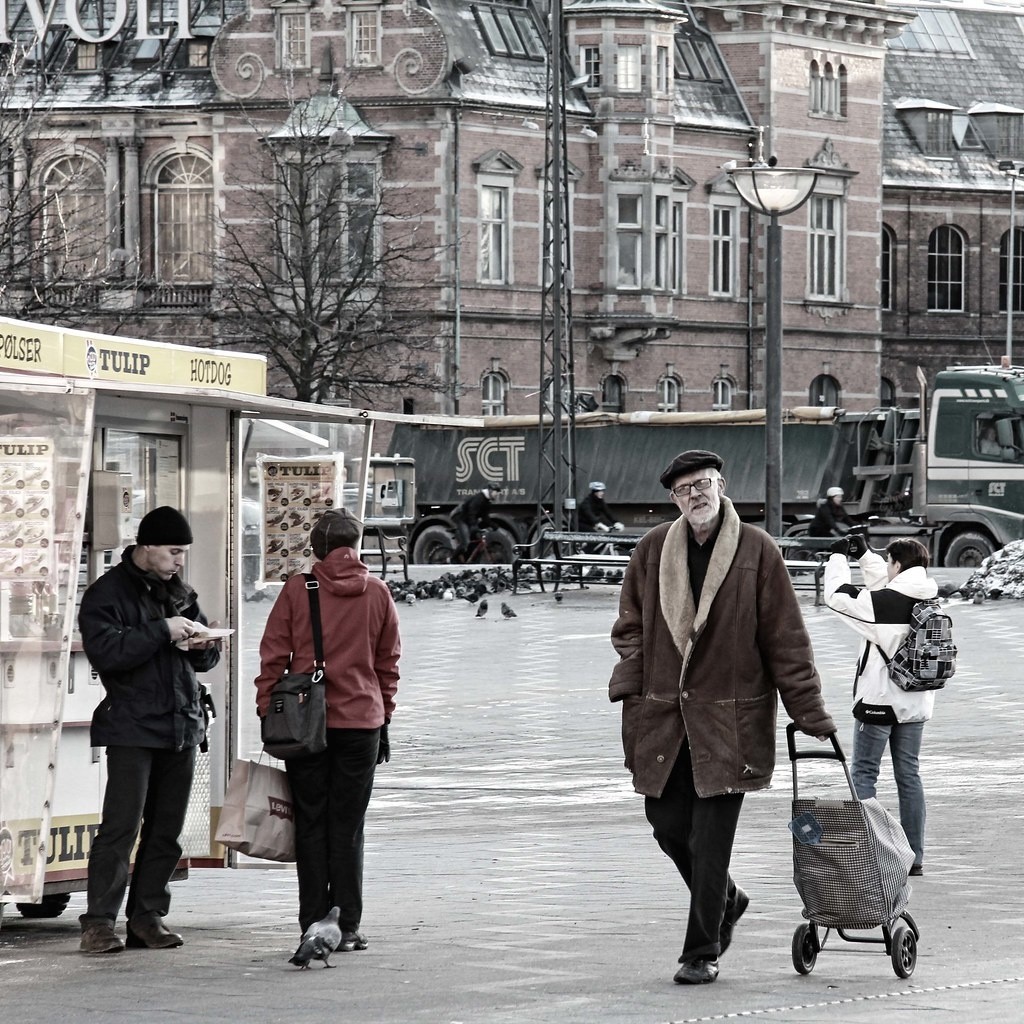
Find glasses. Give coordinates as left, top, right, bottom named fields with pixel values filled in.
left=672, top=477, right=717, bottom=498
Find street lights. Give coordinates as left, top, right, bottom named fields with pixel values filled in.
left=997, top=158, right=1024, bottom=364
left=726, top=155, right=825, bottom=543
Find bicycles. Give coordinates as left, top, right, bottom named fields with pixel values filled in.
left=426, top=528, right=508, bottom=565
left=556, top=526, right=629, bottom=556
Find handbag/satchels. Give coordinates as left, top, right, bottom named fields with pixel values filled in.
left=450, top=506, right=464, bottom=523
left=214, top=747, right=297, bottom=863
left=262, top=673, right=329, bottom=760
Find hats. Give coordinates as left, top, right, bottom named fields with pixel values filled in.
left=309, top=508, right=365, bottom=560
left=660, top=450, right=724, bottom=489
left=136, top=506, right=193, bottom=546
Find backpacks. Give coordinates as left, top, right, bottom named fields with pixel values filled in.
left=876, top=599, right=958, bottom=692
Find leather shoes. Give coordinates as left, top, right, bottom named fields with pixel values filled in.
left=80, top=922, right=124, bottom=954
left=125, top=915, right=183, bottom=949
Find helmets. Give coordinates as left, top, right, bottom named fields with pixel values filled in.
left=589, top=482, right=606, bottom=490
left=827, top=487, right=844, bottom=497
left=488, top=481, right=502, bottom=492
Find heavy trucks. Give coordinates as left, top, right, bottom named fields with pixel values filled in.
left=405, top=351, right=1024, bottom=567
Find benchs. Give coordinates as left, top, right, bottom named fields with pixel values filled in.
left=511, top=528, right=647, bottom=595
left=770, top=534, right=854, bottom=607
left=360, top=525, right=409, bottom=582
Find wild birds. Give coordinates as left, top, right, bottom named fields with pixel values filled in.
left=938, top=583, right=1004, bottom=603
left=717, top=160, right=736, bottom=169
left=475, top=598, right=490, bottom=617
left=386, top=565, right=629, bottom=606
left=500, top=602, right=518, bottom=619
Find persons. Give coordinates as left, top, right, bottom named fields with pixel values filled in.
left=578, top=482, right=625, bottom=554
left=78, top=506, right=220, bottom=956
left=980, top=427, right=1001, bottom=455
left=824, top=533, right=939, bottom=875
left=254, top=508, right=401, bottom=952
left=444, top=482, right=501, bottom=564
left=608, top=451, right=837, bottom=986
left=807, top=487, right=860, bottom=562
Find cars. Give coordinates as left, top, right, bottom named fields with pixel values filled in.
left=242, top=495, right=262, bottom=583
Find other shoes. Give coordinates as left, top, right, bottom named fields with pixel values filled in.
left=454, top=552, right=465, bottom=563
left=908, top=865, right=924, bottom=877
left=340, top=931, right=368, bottom=951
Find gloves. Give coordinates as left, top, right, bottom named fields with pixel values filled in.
left=614, top=522, right=625, bottom=532
left=470, top=526, right=478, bottom=534
left=831, top=538, right=849, bottom=556
left=595, top=523, right=609, bottom=532
left=488, top=522, right=498, bottom=531
left=847, top=534, right=868, bottom=558
left=376, top=721, right=390, bottom=764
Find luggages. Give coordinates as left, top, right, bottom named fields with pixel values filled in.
left=786, top=720, right=919, bottom=978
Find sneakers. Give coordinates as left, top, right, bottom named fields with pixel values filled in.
left=719, top=884, right=749, bottom=957
left=673, top=957, right=719, bottom=984
